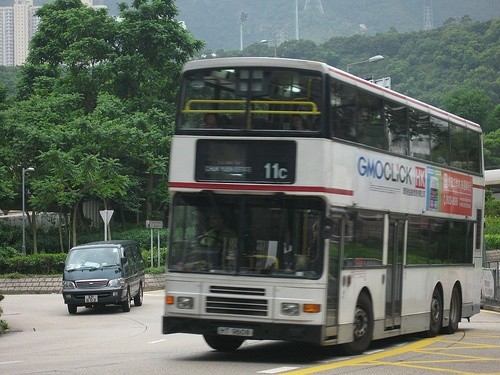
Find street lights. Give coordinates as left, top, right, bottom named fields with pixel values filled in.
left=21, top=166, right=35, bottom=256
left=260, top=38, right=277, bottom=57
left=239, top=10, right=248, bottom=51
left=347, top=54, right=385, bottom=73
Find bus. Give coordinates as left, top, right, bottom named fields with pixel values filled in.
left=160, top=58, right=487, bottom=358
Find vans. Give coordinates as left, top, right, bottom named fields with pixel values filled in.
left=60, top=240, right=146, bottom=315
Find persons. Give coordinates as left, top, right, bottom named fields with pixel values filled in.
left=306, top=222, right=318, bottom=259
left=291, top=115, right=310, bottom=130
left=200, top=212, right=237, bottom=261
left=203, top=113, right=220, bottom=129
